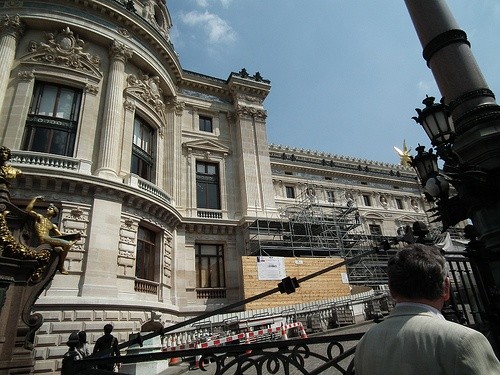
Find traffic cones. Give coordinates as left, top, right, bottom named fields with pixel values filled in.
left=190, top=340, right=209, bottom=370
left=169, top=340, right=182, bottom=365
left=244, top=332, right=252, bottom=355
left=281, top=321, right=289, bottom=341
left=300, top=322, right=308, bottom=339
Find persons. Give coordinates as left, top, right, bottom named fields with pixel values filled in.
left=78, top=331, right=91, bottom=357
left=92, top=324, right=121, bottom=371
left=353, top=244, right=500, bottom=375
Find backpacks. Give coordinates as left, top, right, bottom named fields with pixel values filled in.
left=99, top=337, right=116, bottom=370
